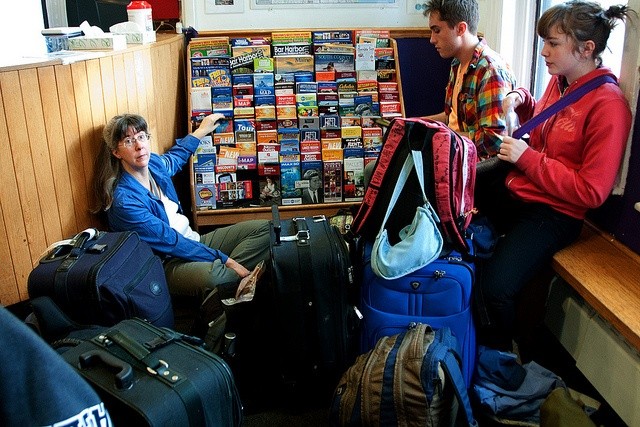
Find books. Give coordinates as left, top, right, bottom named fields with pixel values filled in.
left=190, top=28, right=402, bottom=210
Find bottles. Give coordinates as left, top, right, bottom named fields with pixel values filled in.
left=126, top=0, right=154, bottom=32
left=505, top=105, right=519, bottom=137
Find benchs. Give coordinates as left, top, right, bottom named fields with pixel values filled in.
left=552, top=219, right=639, bottom=349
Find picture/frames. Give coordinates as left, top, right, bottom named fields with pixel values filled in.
left=250, top=0, right=400, bottom=10
left=204, top=0, right=245, bottom=14
left=406, top=0, right=430, bottom=16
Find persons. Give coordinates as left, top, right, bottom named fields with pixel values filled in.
left=302, top=169, right=323, bottom=203
left=476, top=0, right=640, bottom=363
left=361, top=0, right=518, bottom=196
left=261, top=175, right=280, bottom=198
left=345, top=171, right=355, bottom=185
left=88, top=113, right=271, bottom=294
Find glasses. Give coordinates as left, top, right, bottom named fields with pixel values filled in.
left=115, top=134, right=151, bottom=148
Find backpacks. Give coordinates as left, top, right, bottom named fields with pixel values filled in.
left=352, top=116, right=478, bottom=264
left=341, top=325, right=479, bottom=424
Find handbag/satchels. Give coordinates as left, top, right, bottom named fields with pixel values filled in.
left=371, top=203, right=443, bottom=281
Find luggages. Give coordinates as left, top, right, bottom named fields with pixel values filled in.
left=360, top=236, right=475, bottom=380
left=28, top=227, right=175, bottom=336
left=61, top=317, right=245, bottom=427
left=267, top=202, right=355, bottom=391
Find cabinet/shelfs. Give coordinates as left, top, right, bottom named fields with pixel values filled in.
left=186, top=36, right=407, bottom=234
